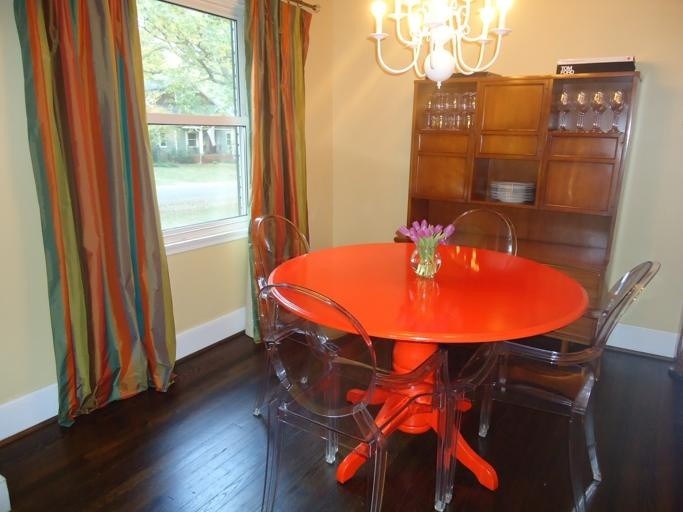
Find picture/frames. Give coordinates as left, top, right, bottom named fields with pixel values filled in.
left=268, top=242, right=587, bottom=494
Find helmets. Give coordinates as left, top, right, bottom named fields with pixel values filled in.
left=490, top=179, right=534, bottom=203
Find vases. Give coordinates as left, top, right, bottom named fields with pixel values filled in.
left=398, top=219, right=455, bottom=276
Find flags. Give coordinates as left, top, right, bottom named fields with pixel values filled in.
left=409, top=247, right=442, bottom=279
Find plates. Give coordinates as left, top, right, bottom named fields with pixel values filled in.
left=406, top=71, right=642, bottom=382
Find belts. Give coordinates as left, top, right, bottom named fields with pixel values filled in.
left=590, top=92, right=606, bottom=134
left=557, top=90, right=571, bottom=130
left=573, top=91, right=588, bottom=132
left=609, top=88, right=626, bottom=135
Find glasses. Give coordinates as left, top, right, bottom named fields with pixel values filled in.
left=368, top=0, right=513, bottom=89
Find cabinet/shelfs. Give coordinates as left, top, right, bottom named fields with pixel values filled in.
left=439, top=115, right=445, bottom=129
left=429, top=93, right=433, bottom=108
left=455, top=114, right=463, bottom=129
left=444, top=93, right=451, bottom=109
left=470, top=93, right=475, bottom=110
left=466, top=115, right=472, bottom=128
left=435, top=93, right=443, bottom=110
left=427, top=114, right=432, bottom=128
left=462, top=93, right=470, bottom=110
left=452, top=95, right=459, bottom=109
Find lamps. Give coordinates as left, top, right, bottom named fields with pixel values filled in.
left=457, top=260, right=662, bottom=512
left=442, top=208, right=518, bottom=256
left=251, top=215, right=354, bottom=464
left=256, top=283, right=448, bottom=510
